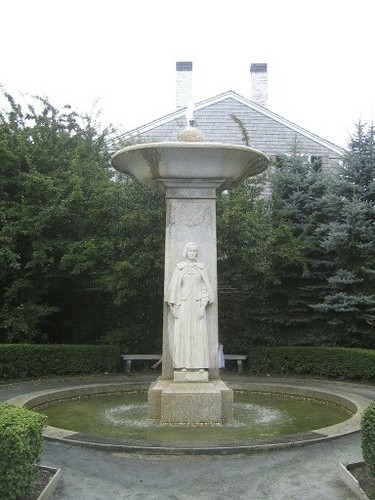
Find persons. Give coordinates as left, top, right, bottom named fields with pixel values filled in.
left=218, top=343, right=225, bottom=377
left=163, top=242, right=216, bottom=373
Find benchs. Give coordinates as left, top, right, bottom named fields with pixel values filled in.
left=120, top=354, right=162, bottom=374
left=222, top=354, right=248, bottom=375
